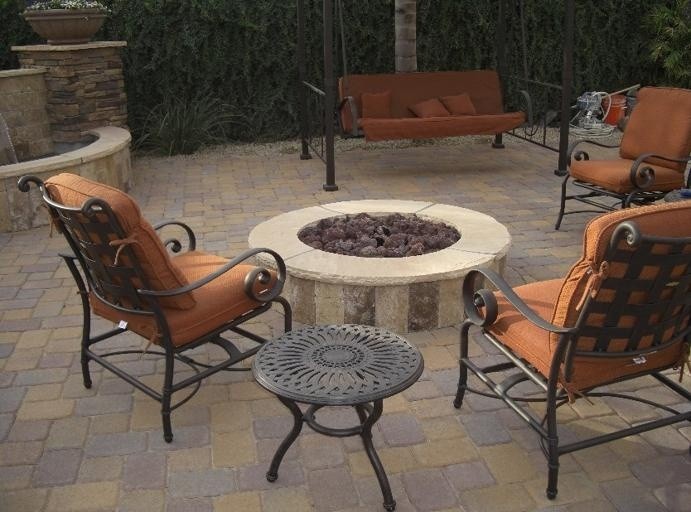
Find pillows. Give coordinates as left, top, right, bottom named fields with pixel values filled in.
left=43, top=171, right=197, bottom=311
left=549, top=200, right=691, bottom=364
left=408, top=99, right=448, bottom=117
left=441, top=93, right=474, bottom=114
left=619, top=86, right=691, bottom=173
left=360, top=89, right=392, bottom=118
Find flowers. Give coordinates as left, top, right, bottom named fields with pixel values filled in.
left=25, top=0, right=114, bottom=16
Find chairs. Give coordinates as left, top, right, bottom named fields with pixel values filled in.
left=453, top=201, right=691, bottom=500
left=16, top=173, right=292, bottom=443
left=556, top=87, right=690, bottom=230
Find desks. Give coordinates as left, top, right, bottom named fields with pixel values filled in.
left=253, top=322, right=425, bottom=512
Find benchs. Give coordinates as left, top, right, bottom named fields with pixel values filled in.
left=339, top=69, right=534, bottom=143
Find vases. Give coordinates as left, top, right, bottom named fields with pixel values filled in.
left=22, top=10, right=110, bottom=44
